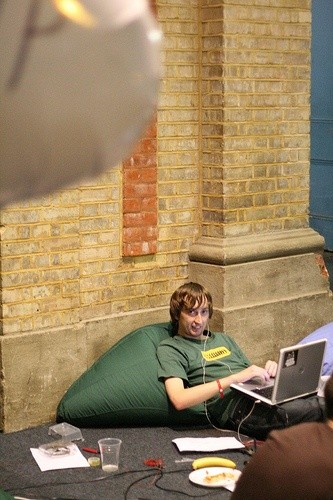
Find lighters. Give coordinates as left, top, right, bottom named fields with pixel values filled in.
left=82, top=447, right=99, bottom=455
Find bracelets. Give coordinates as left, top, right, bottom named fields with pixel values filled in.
left=215, top=379, right=224, bottom=398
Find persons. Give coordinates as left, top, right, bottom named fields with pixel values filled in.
left=155, top=282, right=329, bottom=442
left=230, top=373, right=333, bottom=500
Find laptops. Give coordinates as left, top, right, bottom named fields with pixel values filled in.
left=230, top=340, right=326, bottom=408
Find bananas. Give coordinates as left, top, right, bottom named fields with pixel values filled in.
left=191, top=457, right=236, bottom=470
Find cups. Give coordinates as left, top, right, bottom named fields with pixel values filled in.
left=98, top=437, right=122, bottom=472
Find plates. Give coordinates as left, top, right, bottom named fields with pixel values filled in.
left=189, top=466, right=242, bottom=488
